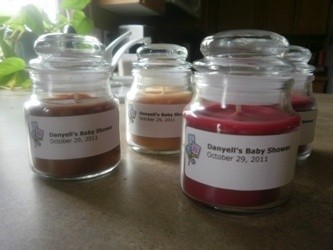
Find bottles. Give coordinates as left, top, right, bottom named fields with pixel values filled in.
left=25, top=33, right=121, bottom=180
left=179, top=29, right=301, bottom=210
left=281, top=45, right=318, bottom=161
left=124, top=42, right=194, bottom=156
